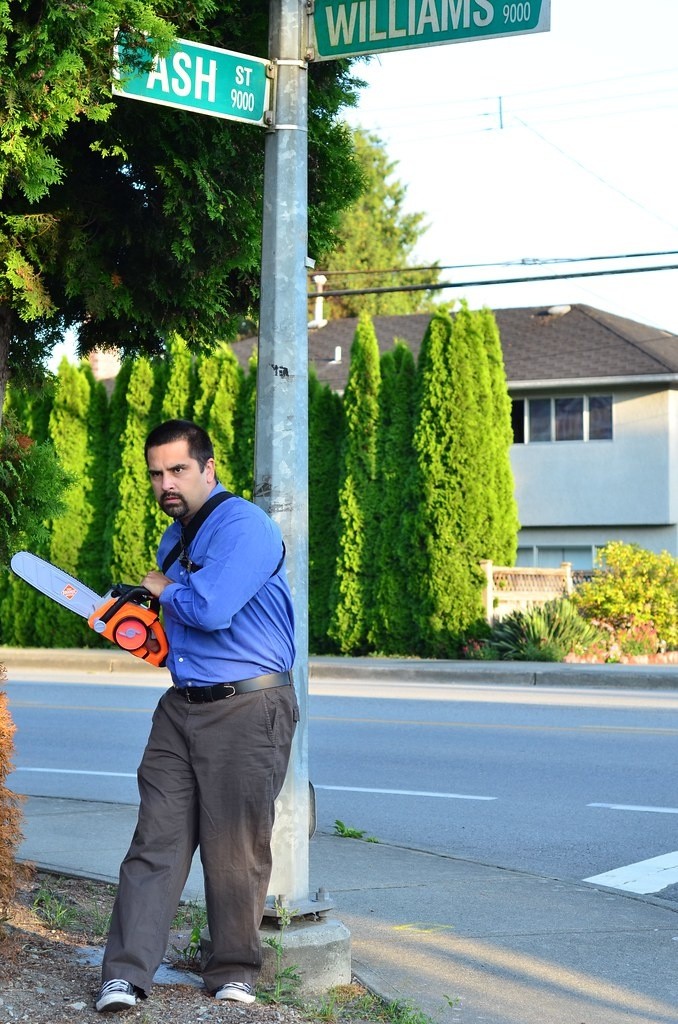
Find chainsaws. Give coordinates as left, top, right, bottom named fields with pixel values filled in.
left=8, top=548, right=171, bottom=668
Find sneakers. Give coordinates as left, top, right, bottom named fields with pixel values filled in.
left=215, top=981, right=257, bottom=1003
left=96, top=979, right=136, bottom=1012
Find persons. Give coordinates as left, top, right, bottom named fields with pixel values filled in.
left=95, top=419, right=300, bottom=1010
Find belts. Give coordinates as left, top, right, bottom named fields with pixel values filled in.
left=173, top=671, right=291, bottom=703
